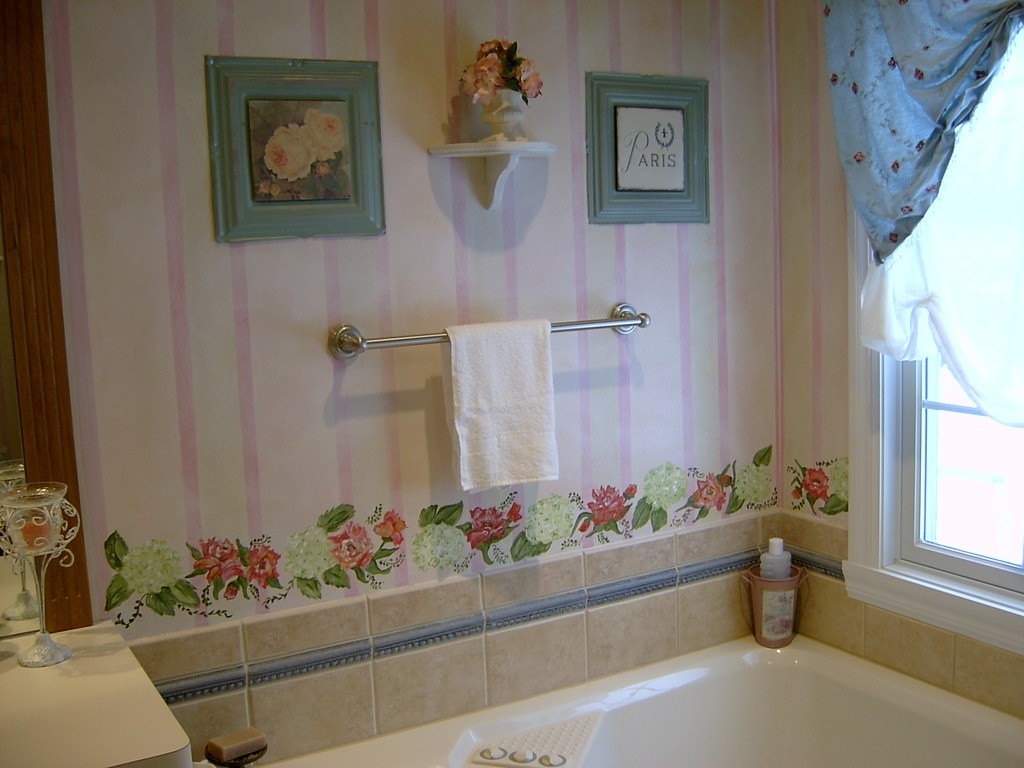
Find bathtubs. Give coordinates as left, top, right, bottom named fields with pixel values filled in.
left=208, top=628, right=1024, bottom=768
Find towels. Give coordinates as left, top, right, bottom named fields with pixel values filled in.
left=437, top=318, right=562, bottom=493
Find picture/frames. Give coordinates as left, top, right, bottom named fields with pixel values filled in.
left=585, top=72, right=712, bottom=226
left=203, top=54, right=387, bottom=243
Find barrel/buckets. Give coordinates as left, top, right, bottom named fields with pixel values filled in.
left=741, top=563, right=809, bottom=650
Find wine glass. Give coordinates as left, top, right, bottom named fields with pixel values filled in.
left=0, top=458, right=38, bottom=621
left=0, top=481, right=72, bottom=669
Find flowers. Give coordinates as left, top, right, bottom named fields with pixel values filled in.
left=459, top=37, right=544, bottom=105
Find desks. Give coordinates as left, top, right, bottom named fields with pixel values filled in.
left=0, top=620, right=195, bottom=768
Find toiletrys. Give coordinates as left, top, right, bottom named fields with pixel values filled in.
left=754, top=532, right=795, bottom=581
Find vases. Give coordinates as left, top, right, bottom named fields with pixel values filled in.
left=480, top=90, right=527, bottom=143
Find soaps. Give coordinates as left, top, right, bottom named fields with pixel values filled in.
left=207, top=725, right=266, bottom=762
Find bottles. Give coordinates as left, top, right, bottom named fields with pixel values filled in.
left=760, top=536, right=793, bottom=578
